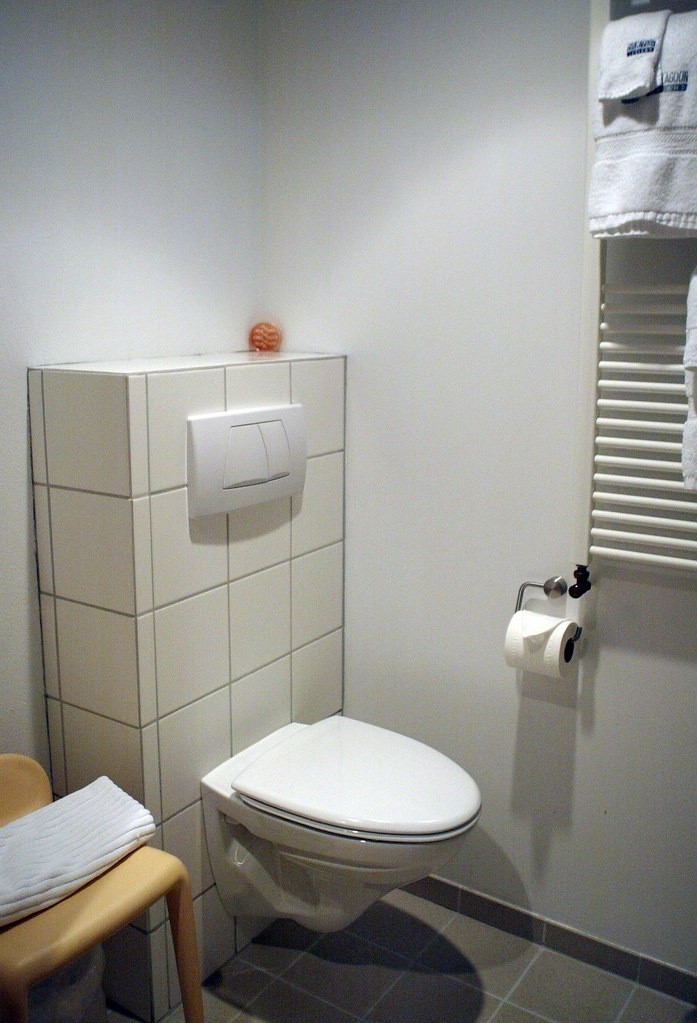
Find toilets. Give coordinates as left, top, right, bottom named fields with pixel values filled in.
left=200, top=712, right=484, bottom=934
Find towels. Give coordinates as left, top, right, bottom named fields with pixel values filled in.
left=680, top=266, right=697, bottom=493
left=586, top=7, right=697, bottom=240
left=597, top=5, right=672, bottom=103
left=0, top=770, right=158, bottom=926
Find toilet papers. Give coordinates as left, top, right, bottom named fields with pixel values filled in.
left=504, top=608, right=579, bottom=680
